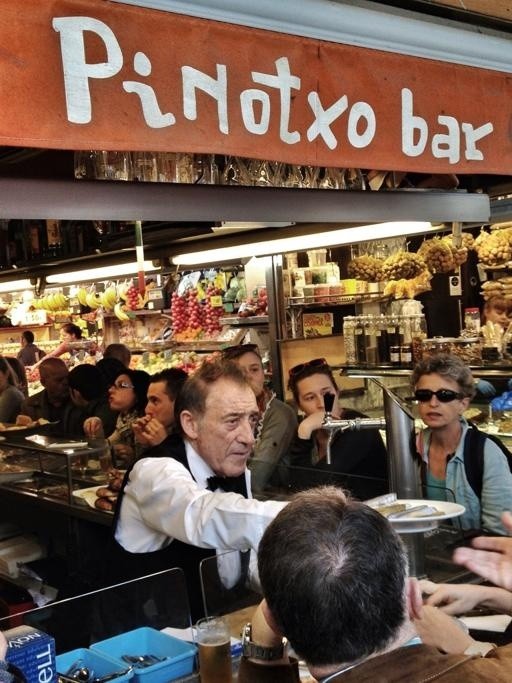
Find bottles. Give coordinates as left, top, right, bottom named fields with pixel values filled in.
left=479, top=338, right=501, bottom=360
left=285, top=249, right=389, bottom=303
left=342, top=307, right=481, bottom=364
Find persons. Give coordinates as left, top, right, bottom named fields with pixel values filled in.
left=425, top=583, right=512, bottom=616
left=287, top=358, right=390, bottom=503
left=220, top=344, right=299, bottom=495
left=453, top=510, right=512, bottom=591
left=100, top=359, right=293, bottom=631
left=0, top=306, right=190, bottom=469
left=411, top=356, right=512, bottom=536
left=237, top=484, right=512, bottom=683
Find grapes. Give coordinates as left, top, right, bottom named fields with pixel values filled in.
left=170, top=283, right=224, bottom=336
left=124, top=285, right=140, bottom=312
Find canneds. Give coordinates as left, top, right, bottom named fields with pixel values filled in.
left=400, top=345, right=412, bottom=367
left=464, top=307, right=481, bottom=332
left=389, top=345, right=400, bottom=366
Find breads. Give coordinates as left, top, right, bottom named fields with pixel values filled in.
left=94, top=476, right=122, bottom=511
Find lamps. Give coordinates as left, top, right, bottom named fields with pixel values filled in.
left=169, top=220, right=448, bottom=266
left=44, top=258, right=165, bottom=284
left=0, top=271, right=44, bottom=295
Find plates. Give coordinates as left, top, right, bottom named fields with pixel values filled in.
left=377, top=498, right=466, bottom=533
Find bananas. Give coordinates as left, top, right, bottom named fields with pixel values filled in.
left=101, top=287, right=117, bottom=311
left=113, top=301, right=130, bottom=322
left=39, top=289, right=70, bottom=313
left=85, top=289, right=101, bottom=310
left=119, top=289, right=128, bottom=301
left=76, top=285, right=88, bottom=307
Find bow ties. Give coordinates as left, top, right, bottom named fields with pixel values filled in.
left=206, top=474, right=237, bottom=493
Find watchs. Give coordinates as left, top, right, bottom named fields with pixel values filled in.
left=240, top=623, right=284, bottom=661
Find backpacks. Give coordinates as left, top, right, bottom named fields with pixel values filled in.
left=463, top=419, right=512, bottom=501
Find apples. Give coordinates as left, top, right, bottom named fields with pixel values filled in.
left=236, top=285, right=268, bottom=318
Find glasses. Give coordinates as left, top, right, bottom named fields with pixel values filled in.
left=287, top=356, right=327, bottom=377
left=114, top=381, right=136, bottom=391
left=222, top=343, right=258, bottom=358
left=414, top=389, right=464, bottom=403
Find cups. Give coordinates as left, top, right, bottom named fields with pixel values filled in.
left=197, top=616, right=232, bottom=683
